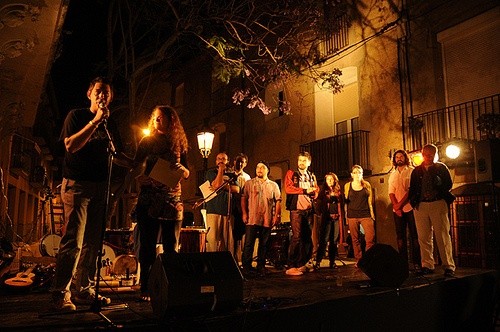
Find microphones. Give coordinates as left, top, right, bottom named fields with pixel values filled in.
left=98, top=103, right=107, bottom=122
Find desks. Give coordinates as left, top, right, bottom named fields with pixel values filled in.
left=105, top=228, right=207, bottom=253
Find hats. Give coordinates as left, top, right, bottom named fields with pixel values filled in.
left=256, top=160, right=270, bottom=175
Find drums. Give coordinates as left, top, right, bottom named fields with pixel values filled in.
left=181, top=228, right=208, bottom=253
left=95, top=244, right=115, bottom=279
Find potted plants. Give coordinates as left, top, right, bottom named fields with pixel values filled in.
left=475, top=113, right=500, bottom=140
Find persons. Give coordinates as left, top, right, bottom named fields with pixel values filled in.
left=389, top=150, right=422, bottom=274
left=203, top=151, right=281, bottom=277
left=409, top=144, right=456, bottom=277
left=344, top=164, right=375, bottom=268
left=314, top=172, right=341, bottom=271
left=50, top=77, right=114, bottom=314
left=284, top=150, right=319, bottom=275
left=126, top=105, right=190, bottom=304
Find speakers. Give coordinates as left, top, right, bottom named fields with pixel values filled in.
left=150, top=250, right=243, bottom=319
left=356, top=243, right=408, bottom=290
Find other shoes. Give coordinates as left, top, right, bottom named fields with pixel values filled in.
left=444, top=268, right=455, bottom=277
left=328, top=262, right=340, bottom=271
left=243, top=266, right=257, bottom=273
left=415, top=267, right=434, bottom=275
left=285, top=266, right=307, bottom=275
left=314, top=262, right=320, bottom=271
left=256, top=266, right=271, bottom=273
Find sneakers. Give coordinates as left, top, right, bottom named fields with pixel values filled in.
left=47, top=289, right=76, bottom=312
left=75, top=282, right=111, bottom=306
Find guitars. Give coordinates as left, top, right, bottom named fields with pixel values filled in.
left=4, top=263, right=37, bottom=291
left=39, top=185, right=62, bottom=257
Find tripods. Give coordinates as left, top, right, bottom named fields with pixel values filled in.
left=37, top=119, right=130, bottom=329
